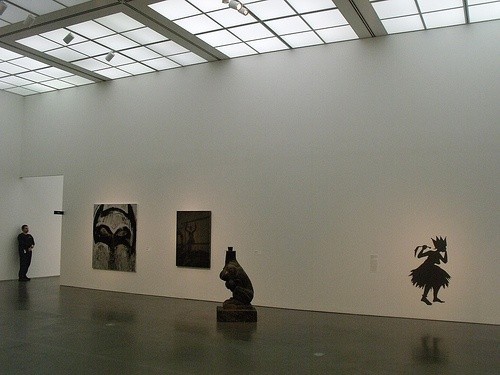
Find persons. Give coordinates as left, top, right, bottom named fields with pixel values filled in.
left=17, top=224, right=35, bottom=282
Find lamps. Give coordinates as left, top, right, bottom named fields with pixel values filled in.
left=238, top=6, right=249, bottom=16
left=229, top=0, right=242, bottom=10
left=23, top=14, right=35, bottom=28
left=105, top=52, right=115, bottom=62
left=63, top=32, right=74, bottom=44
left=222, top=0, right=229, bottom=4
left=0, top=2, right=7, bottom=15
